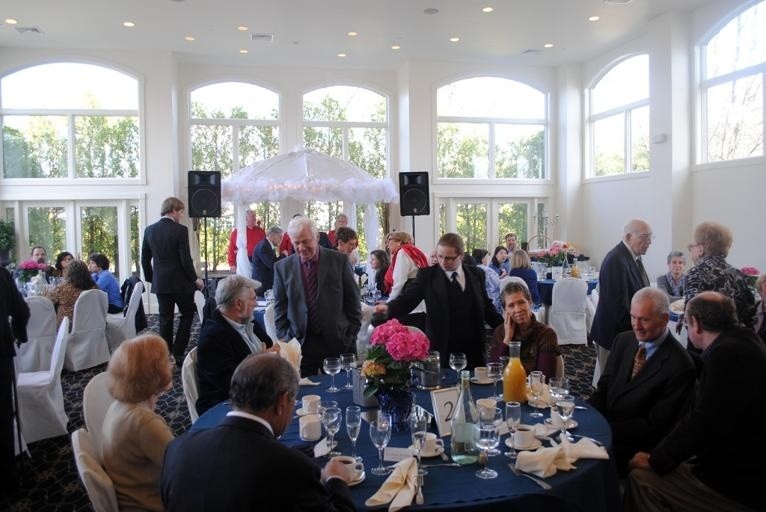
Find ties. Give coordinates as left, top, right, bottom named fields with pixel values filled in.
left=450, top=272, right=464, bottom=292
left=631, top=343, right=648, bottom=380
left=305, top=259, right=320, bottom=335
left=636, top=257, right=650, bottom=287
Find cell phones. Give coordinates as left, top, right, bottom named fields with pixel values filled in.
left=501, top=306, right=506, bottom=315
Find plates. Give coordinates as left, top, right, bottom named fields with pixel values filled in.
left=544, top=418, right=578, bottom=429
left=296, top=407, right=327, bottom=416
left=505, top=437, right=542, bottom=450
left=408, top=445, right=444, bottom=457
left=470, top=377, right=494, bottom=384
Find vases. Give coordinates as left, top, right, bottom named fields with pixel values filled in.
left=376, top=385, right=415, bottom=422
left=19, top=283, right=27, bottom=297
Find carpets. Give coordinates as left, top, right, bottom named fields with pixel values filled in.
left=0, top=314, right=598, bottom=512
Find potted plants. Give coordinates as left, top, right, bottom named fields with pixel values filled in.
left=0, top=221, right=17, bottom=265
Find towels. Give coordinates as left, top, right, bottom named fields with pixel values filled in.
left=550, top=434, right=608, bottom=461
left=365, top=457, right=419, bottom=512
left=360, top=274, right=368, bottom=288
left=515, top=447, right=576, bottom=478
left=272, top=337, right=320, bottom=386
left=524, top=382, right=556, bottom=407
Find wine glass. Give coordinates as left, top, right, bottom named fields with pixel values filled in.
left=370, top=413, right=392, bottom=476
left=479, top=407, right=503, bottom=457
left=556, top=395, right=575, bottom=442
left=322, top=407, right=342, bottom=458
left=449, top=353, right=467, bottom=387
left=499, top=356, right=509, bottom=367
left=411, top=416, right=428, bottom=476
left=369, top=290, right=381, bottom=301
left=487, top=362, right=503, bottom=402
left=529, top=371, right=544, bottom=418
left=346, top=405, right=363, bottom=462
left=340, top=353, right=355, bottom=390
left=49, top=276, right=54, bottom=284
left=316, top=400, right=338, bottom=446
left=473, top=424, right=500, bottom=480
left=549, top=377, right=570, bottom=399
left=323, top=357, right=341, bottom=393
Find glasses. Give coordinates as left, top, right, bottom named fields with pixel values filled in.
left=688, top=244, right=702, bottom=252
left=640, top=234, right=653, bottom=239
left=386, top=238, right=399, bottom=244
left=437, top=254, right=462, bottom=263
left=167, top=355, right=176, bottom=366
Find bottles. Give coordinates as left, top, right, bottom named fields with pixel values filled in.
left=503, top=340, right=528, bottom=403
left=562, top=252, right=571, bottom=278
left=39, top=269, right=49, bottom=297
left=450, top=371, right=481, bottom=465
left=570, top=258, right=581, bottom=278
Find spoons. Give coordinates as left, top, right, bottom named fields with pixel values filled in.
left=504, top=401, right=521, bottom=460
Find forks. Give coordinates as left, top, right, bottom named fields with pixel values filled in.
left=507, top=463, right=552, bottom=490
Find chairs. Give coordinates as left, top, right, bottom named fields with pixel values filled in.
left=500, top=276, right=546, bottom=324
left=264, top=304, right=289, bottom=344
left=63, top=289, right=111, bottom=372
left=194, top=290, right=205, bottom=324
left=536, top=277, right=588, bottom=347
left=13, top=316, right=69, bottom=443
left=106, top=275, right=139, bottom=317
left=83, top=372, right=116, bottom=463
left=181, top=347, right=198, bottom=424
left=106, top=282, right=142, bottom=355
left=71, top=428, right=118, bottom=512
left=668, top=321, right=688, bottom=350
left=13, top=296, right=58, bottom=373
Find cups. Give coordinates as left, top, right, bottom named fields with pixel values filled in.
left=515, top=425, right=536, bottom=447
left=302, top=395, right=321, bottom=414
left=475, top=367, right=488, bottom=382
left=476, top=399, right=501, bottom=420
left=299, top=415, right=320, bottom=441
left=551, top=407, right=562, bottom=425
left=413, top=432, right=443, bottom=454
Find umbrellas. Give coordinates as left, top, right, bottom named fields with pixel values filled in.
left=220, top=148, right=399, bottom=218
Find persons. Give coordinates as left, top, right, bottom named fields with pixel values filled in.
left=676, top=221, right=764, bottom=363
left=49, top=252, right=75, bottom=277
left=492, top=250, right=543, bottom=315
left=141, top=198, right=203, bottom=368
left=583, top=287, right=699, bottom=481
left=228, top=211, right=266, bottom=274
left=194, top=275, right=280, bottom=417
left=332, top=227, right=356, bottom=256
left=376, top=231, right=430, bottom=335
left=98, top=334, right=176, bottom=512
left=491, top=277, right=557, bottom=387
left=657, top=251, right=688, bottom=302
left=318, top=232, right=339, bottom=251
left=0, top=266, right=31, bottom=512
left=504, top=233, right=522, bottom=264
left=588, top=219, right=653, bottom=377
left=329, top=214, right=348, bottom=247
left=520, top=242, right=529, bottom=252
left=160, top=353, right=356, bottom=512
left=251, top=228, right=283, bottom=297
left=44, top=259, right=100, bottom=333
left=32, top=247, right=46, bottom=264
left=273, top=216, right=362, bottom=379
left=488, top=246, right=510, bottom=276
left=370, top=250, right=390, bottom=297
left=625, top=291, right=766, bottom=512
left=90, top=255, right=124, bottom=314
left=374, top=233, right=507, bottom=372
left=279, top=213, right=301, bottom=256
left=461, top=255, right=486, bottom=290
left=472, top=249, right=502, bottom=315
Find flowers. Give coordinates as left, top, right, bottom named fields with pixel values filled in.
left=536, top=240, right=576, bottom=267
left=740, top=267, right=760, bottom=291
left=357, top=318, right=429, bottom=399
left=16, top=260, right=47, bottom=283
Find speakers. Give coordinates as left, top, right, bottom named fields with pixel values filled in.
left=399, top=172, right=430, bottom=216
left=188, top=171, right=221, bottom=217
